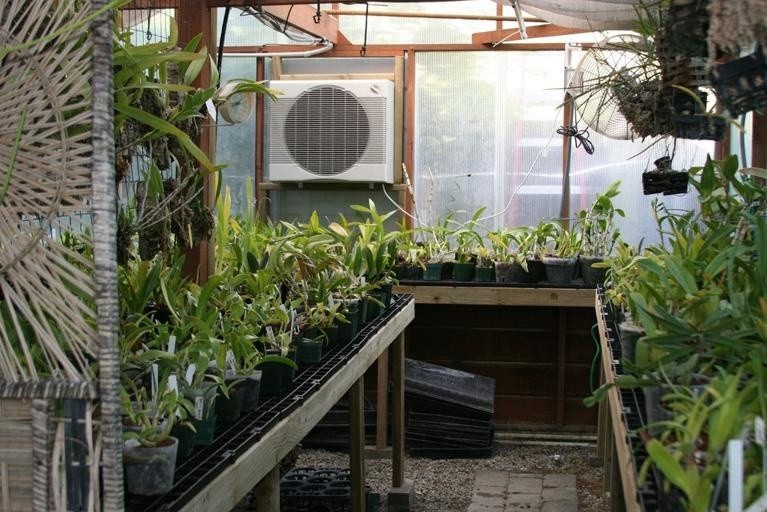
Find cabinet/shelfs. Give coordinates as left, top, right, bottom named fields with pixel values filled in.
left=594, top=284, right=678, bottom=511
left=374, top=278, right=602, bottom=448
left=126, top=292, right=415, bottom=511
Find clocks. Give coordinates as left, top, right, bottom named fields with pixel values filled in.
left=221, top=82, right=255, bottom=124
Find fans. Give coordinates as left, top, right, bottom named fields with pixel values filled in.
left=573, top=33, right=664, bottom=140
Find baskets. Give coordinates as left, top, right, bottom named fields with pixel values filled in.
left=653, top=0, right=767, bottom=141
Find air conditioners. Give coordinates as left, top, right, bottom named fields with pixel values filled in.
left=269, top=80, right=394, bottom=185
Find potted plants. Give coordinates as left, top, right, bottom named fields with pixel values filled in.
left=119, top=197, right=399, bottom=498
left=607, top=182, right=766, bottom=511
left=394, top=182, right=625, bottom=288
